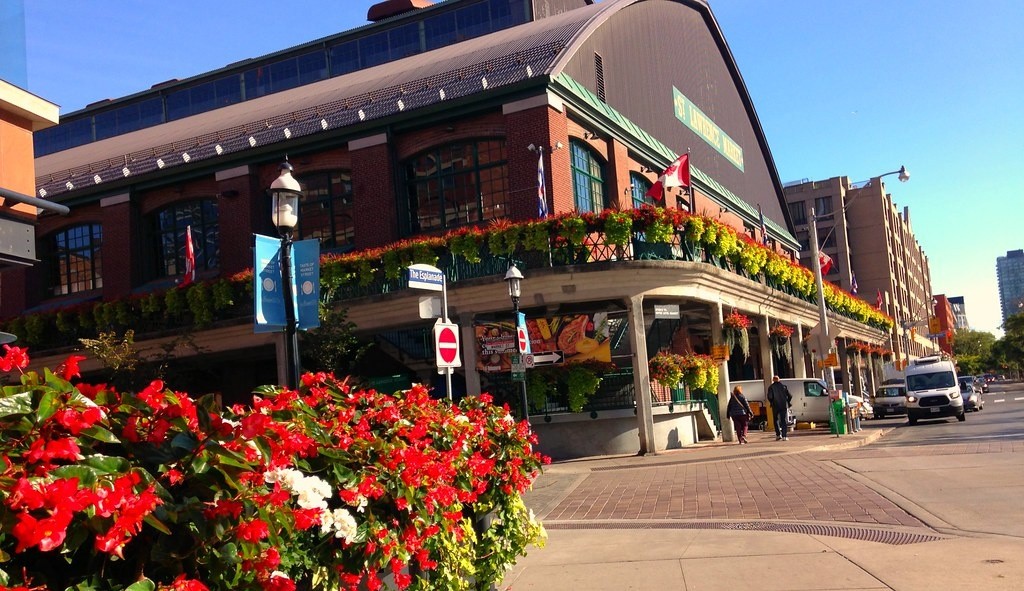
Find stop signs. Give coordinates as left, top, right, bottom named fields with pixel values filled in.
left=439, top=328, right=457, bottom=363
left=519, top=330, right=527, bottom=351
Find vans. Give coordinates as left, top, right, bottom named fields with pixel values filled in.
left=729, top=377, right=873, bottom=431
left=903, top=356, right=967, bottom=426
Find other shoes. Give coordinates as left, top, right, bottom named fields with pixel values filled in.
left=782, top=437, right=789, bottom=441
left=742, top=437, right=748, bottom=444
left=776, top=434, right=782, bottom=441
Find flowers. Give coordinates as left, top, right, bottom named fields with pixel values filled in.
left=0, top=341, right=553, bottom=591
left=769, top=324, right=794, bottom=369
left=721, top=309, right=752, bottom=364
left=512, top=356, right=620, bottom=416
left=0, top=200, right=895, bottom=348
left=847, top=341, right=892, bottom=361
left=648, top=348, right=720, bottom=394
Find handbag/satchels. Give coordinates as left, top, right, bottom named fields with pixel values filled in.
left=746, top=413, right=751, bottom=422
left=786, top=398, right=792, bottom=408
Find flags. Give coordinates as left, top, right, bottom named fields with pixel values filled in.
left=850, top=271, right=859, bottom=296
left=537, top=149, right=550, bottom=220
left=876, top=288, right=883, bottom=310
left=179, top=227, right=196, bottom=287
left=645, top=152, right=690, bottom=200
left=758, top=204, right=767, bottom=246
left=820, top=250, right=833, bottom=276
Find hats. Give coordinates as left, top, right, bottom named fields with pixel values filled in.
left=773, top=376, right=779, bottom=381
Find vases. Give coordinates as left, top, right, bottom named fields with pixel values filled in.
left=778, top=336, right=788, bottom=346
left=724, top=330, right=742, bottom=338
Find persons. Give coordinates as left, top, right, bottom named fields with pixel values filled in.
left=767, top=375, right=792, bottom=441
left=726, top=386, right=754, bottom=445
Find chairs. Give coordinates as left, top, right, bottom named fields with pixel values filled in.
left=915, top=378, right=922, bottom=383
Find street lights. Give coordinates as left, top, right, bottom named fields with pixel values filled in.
left=503, top=262, right=530, bottom=423
left=271, top=154, right=302, bottom=389
left=902, top=298, right=938, bottom=365
left=807, top=166, right=910, bottom=391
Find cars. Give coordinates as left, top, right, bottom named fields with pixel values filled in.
left=871, top=382, right=907, bottom=419
left=956, top=373, right=1006, bottom=413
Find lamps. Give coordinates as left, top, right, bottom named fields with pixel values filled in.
left=585, top=131, right=600, bottom=140
left=745, top=228, right=753, bottom=231
left=641, top=165, right=652, bottom=173
left=680, top=190, right=690, bottom=195
left=784, top=250, right=792, bottom=254
left=720, top=207, right=731, bottom=212
left=767, top=236, right=772, bottom=243
